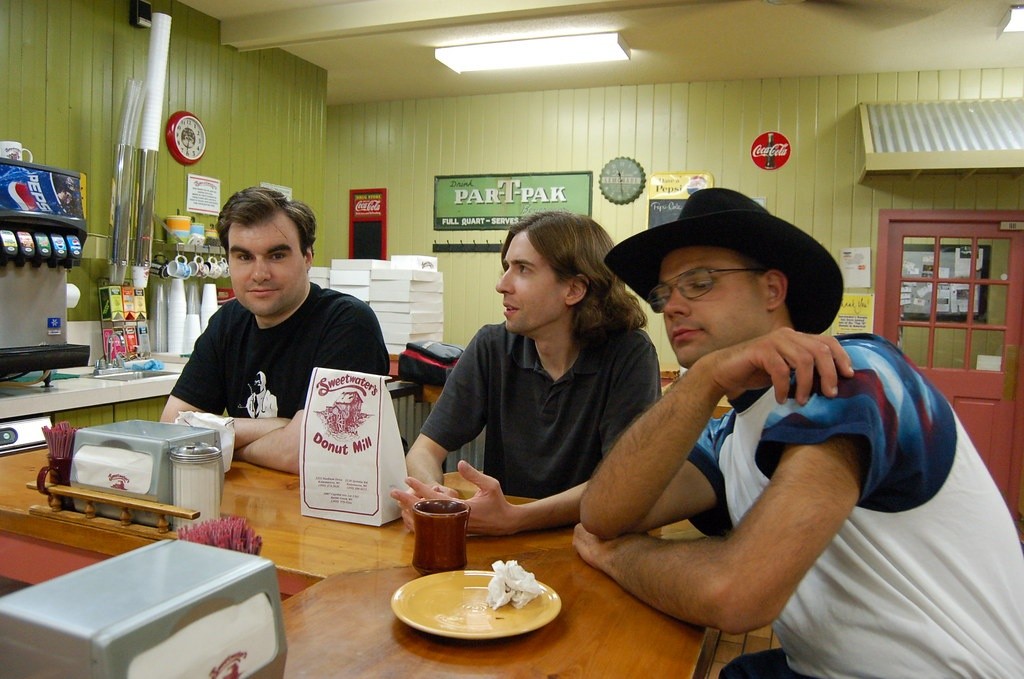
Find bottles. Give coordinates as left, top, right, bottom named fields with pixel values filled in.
left=766, top=134, right=775, bottom=167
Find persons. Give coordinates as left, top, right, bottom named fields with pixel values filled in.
left=574, top=188, right=1024, bottom=679
left=391, top=211, right=662, bottom=536
left=159, top=187, right=408, bottom=476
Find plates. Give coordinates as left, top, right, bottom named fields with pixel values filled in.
left=390, top=570, right=562, bottom=640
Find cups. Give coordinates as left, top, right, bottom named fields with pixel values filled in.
left=150, top=279, right=218, bottom=354
left=132, top=265, right=150, bottom=289
left=137, top=12, right=173, bottom=152
left=150, top=255, right=170, bottom=279
left=205, top=257, right=222, bottom=280
left=218, top=258, right=231, bottom=278
left=412, top=499, right=471, bottom=575
left=0, top=140, right=33, bottom=163
left=37, top=453, right=74, bottom=510
left=169, top=443, right=222, bottom=533
left=188, top=256, right=209, bottom=278
left=108, top=263, right=126, bottom=285
left=114, top=76, right=146, bottom=147
left=167, top=255, right=192, bottom=280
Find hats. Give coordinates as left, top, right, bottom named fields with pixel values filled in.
left=603, top=188, right=844, bottom=335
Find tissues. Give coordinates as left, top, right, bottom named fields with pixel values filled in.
left=69, top=419, right=225, bottom=528
left=1, top=537, right=288, bottom=679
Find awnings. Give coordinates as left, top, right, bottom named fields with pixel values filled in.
left=856, top=97, right=1023, bottom=182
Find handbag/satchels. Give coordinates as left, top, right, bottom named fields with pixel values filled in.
left=398, top=340, right=465, bottom=389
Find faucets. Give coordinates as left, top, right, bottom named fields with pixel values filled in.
left=107, top=332, right=126, bottom=368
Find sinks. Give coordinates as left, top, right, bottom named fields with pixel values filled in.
left=86, top=369, right=182, bottom=382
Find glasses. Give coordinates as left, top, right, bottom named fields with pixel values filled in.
left=648, top=265, right=768, bottom=314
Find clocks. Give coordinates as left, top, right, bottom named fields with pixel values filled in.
left=599, top=157, right=646, bottom=205
left=166, top=111, right=206, bottom=164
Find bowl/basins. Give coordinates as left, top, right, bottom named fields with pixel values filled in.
left=166, top=215, right=219, bottom=245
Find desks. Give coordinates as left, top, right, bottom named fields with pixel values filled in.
left=1, top=443, right=709, bottom=678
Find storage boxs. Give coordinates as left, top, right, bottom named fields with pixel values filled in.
left=308, top=256, right=443, bottom=354
